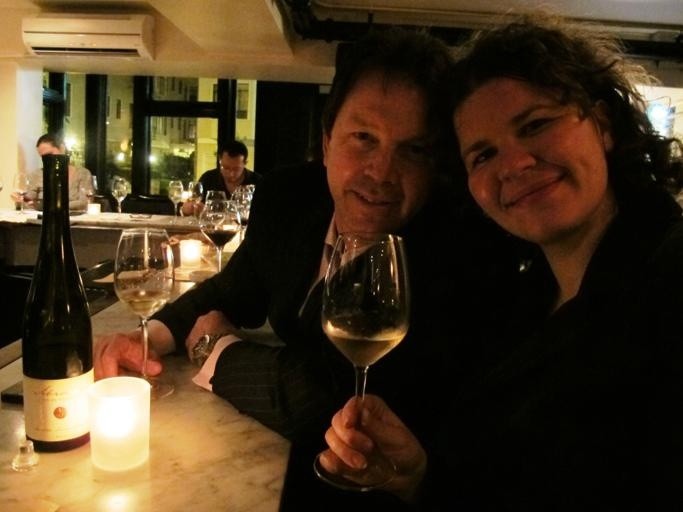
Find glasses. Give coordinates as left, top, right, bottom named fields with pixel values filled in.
left=220, top=159, right=243, bottom=172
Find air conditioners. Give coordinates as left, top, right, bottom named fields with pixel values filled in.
left=20, top=13, right=156, bottom=63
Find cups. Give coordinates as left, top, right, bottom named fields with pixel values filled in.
left=92, top=376, right=152, bottom=471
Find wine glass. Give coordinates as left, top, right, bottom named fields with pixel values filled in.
left=314, top=232, right=411, bottom=492
left=199, top=200, right=241, bottom=273
left=115, top=227, right=174, bottom=400
left=12, top=170, right=255, bottom=224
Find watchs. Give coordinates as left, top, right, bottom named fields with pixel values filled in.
left=190, top=329, right=246, bottom=367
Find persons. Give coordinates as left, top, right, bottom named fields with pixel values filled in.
left=93, top=30, right=477, bottom=454
left=182, top=140, right=252, bottom=216
left=319, top=12, right=683, bottom=512
left=10, top=133, right=94, bottom=214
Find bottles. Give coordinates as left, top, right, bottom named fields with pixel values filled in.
left=21, top=155, right=95, bottom=453
